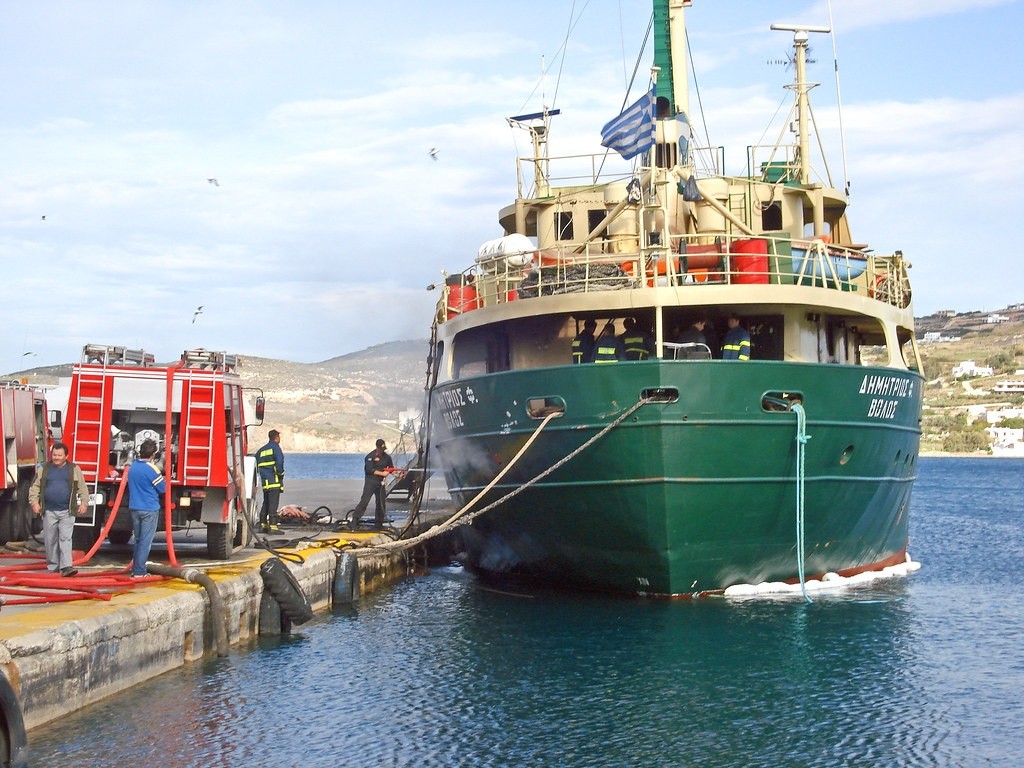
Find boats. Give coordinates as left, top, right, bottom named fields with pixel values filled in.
left=427, top=0, right=930, bottom=596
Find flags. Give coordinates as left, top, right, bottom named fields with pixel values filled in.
left=600, top=85, right=657, bottom=160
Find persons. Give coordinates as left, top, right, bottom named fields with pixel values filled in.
left=128, top=439, right=166, bottom=579
left=255, top=430, right=286, bottom=536
left=572, top=316, right=774, bottom=364
left=349, top=439, right=406, bottom=532
left=28, top=442, right=90, bottom=577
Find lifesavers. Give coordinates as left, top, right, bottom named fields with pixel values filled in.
left=259, top=590, right=292, bottom=635
left=868, top=271, right=889, bottom=297
left=333, top=553, right=360, bottom=604
left=260, top=558, right=313, bottom=625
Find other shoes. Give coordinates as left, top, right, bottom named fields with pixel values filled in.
left=350, top=518, right=358, bottom=530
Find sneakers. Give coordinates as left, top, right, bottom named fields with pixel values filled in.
left=61, top=566, right=78, bottom=577
left=131, top=573, right=152, bottom=578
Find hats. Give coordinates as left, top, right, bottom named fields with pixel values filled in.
left=269, top=430, right=280, bottom=438
left=376, top=439, right=387, bottom=450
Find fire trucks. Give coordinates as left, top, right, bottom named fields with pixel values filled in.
left=60, top=342, right=266, bottom=562
left=0, top=379, right=65, bottom=543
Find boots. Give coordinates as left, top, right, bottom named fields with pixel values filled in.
left=269, top=515, right=284, bottom=535
left=259, top=513, right=269, bottom=532
